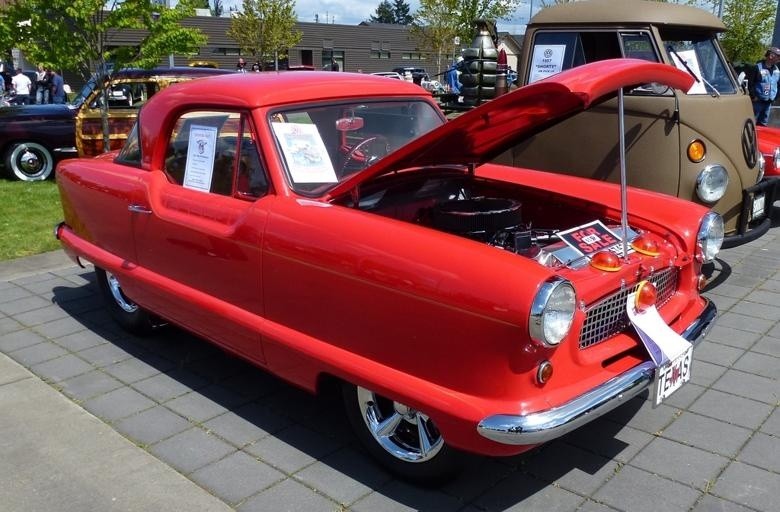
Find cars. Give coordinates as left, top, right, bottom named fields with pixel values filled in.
left=754, top=126, right=780, bottom=215
left=53, top=56, right=725, bottom=489
left=370, top=67, right=429, bottom=85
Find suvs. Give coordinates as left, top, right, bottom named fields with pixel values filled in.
left=437, top=2, right=779, bottom=250
left=1, top=66, right=290, bottom=182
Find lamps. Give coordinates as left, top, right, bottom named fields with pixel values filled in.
left=164, top=113, right=351, bottom=198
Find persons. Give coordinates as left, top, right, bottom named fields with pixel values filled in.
left=237, top=58, right=248, bottom=74
left=331, top=57, right=339, bottom=71
left=0, top=63, right=66, bottom=103
left=749, top=47, right=780, bottom=125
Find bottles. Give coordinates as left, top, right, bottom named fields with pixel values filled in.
left=768, top=47, right=780, bottom=56
